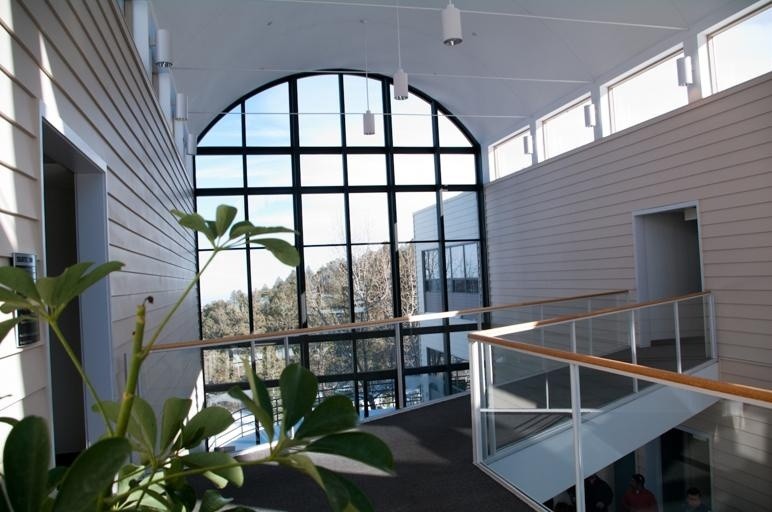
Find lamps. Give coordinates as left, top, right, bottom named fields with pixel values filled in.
left=156, top=25, right=197, bottom=156
left=441, top=1, right=463, bottom=47
left=360, top=17, right=375, bottom=135
left=584, top=104, right=596, bottom=128
left=524, top=136, right=533, bottom=154
left=394, top=1, right=408, bottom=100
left=676, top=56, right=692, bottom=86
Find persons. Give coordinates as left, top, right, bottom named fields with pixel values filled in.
left=683, top=485, right=710, bottom=512
left=572, top=473, right=613, bottom=512
left=618, top=472, right=660, bottom=511
